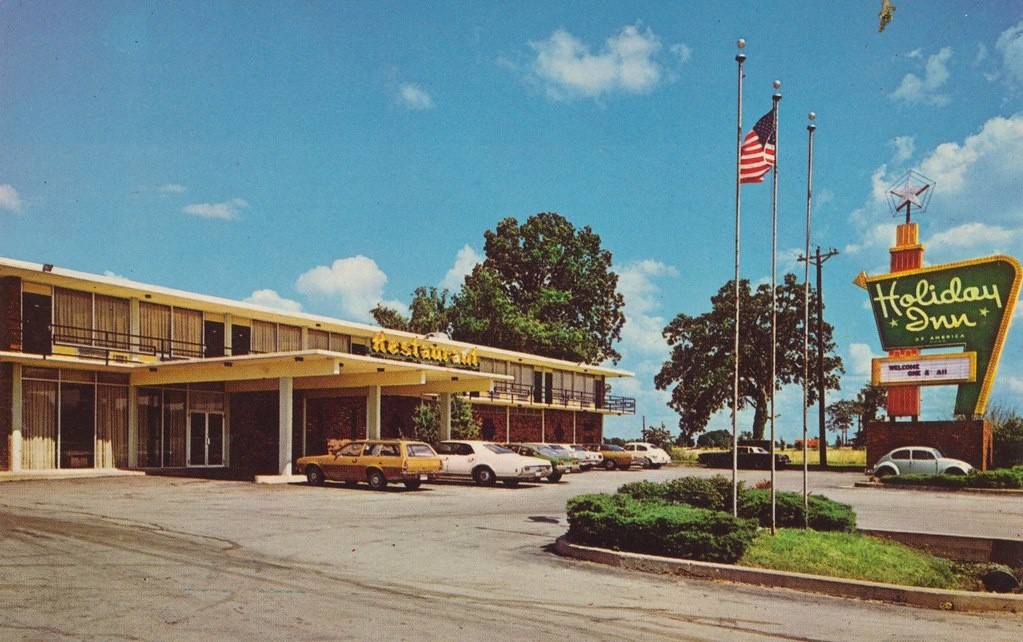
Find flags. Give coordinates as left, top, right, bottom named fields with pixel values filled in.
left=738, top=108, right=778, bottom=183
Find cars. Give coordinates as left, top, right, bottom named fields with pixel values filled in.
left=870, top=446, right=974, bottom=481
left=410, top=440, right=630, bottom=487
left=295, top=439, right=442, bottom=490
left=622, top=442, right=671, bottom=470
left=729, top=447, right=768, bottom=454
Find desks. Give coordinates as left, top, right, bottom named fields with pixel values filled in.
left=63, top=450, right=94, bottom=468
